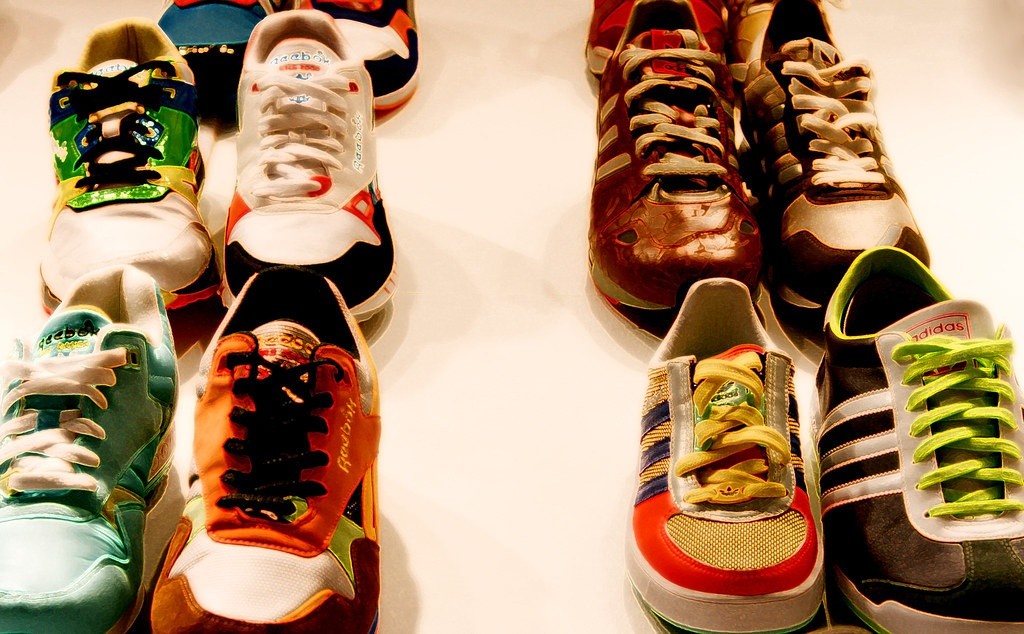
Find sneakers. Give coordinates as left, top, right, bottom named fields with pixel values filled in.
left=219, top=10, right=399, bottom=323
left=293, top=0, right=419, bottom=110
left=155, top=0, right=296, bottom=137
left=0, top=262, right=179, bottom=634
left=150, top=265, right=380, bottom=634
left=624, top=277, right=825, bottom=634
left=742, top=0, right=931, bottom=308
left=810, top=246, right=1024, bottom=634
left=41, top=16, right=220, bottom=318
left=587, top=0, right=763, bottom=342
